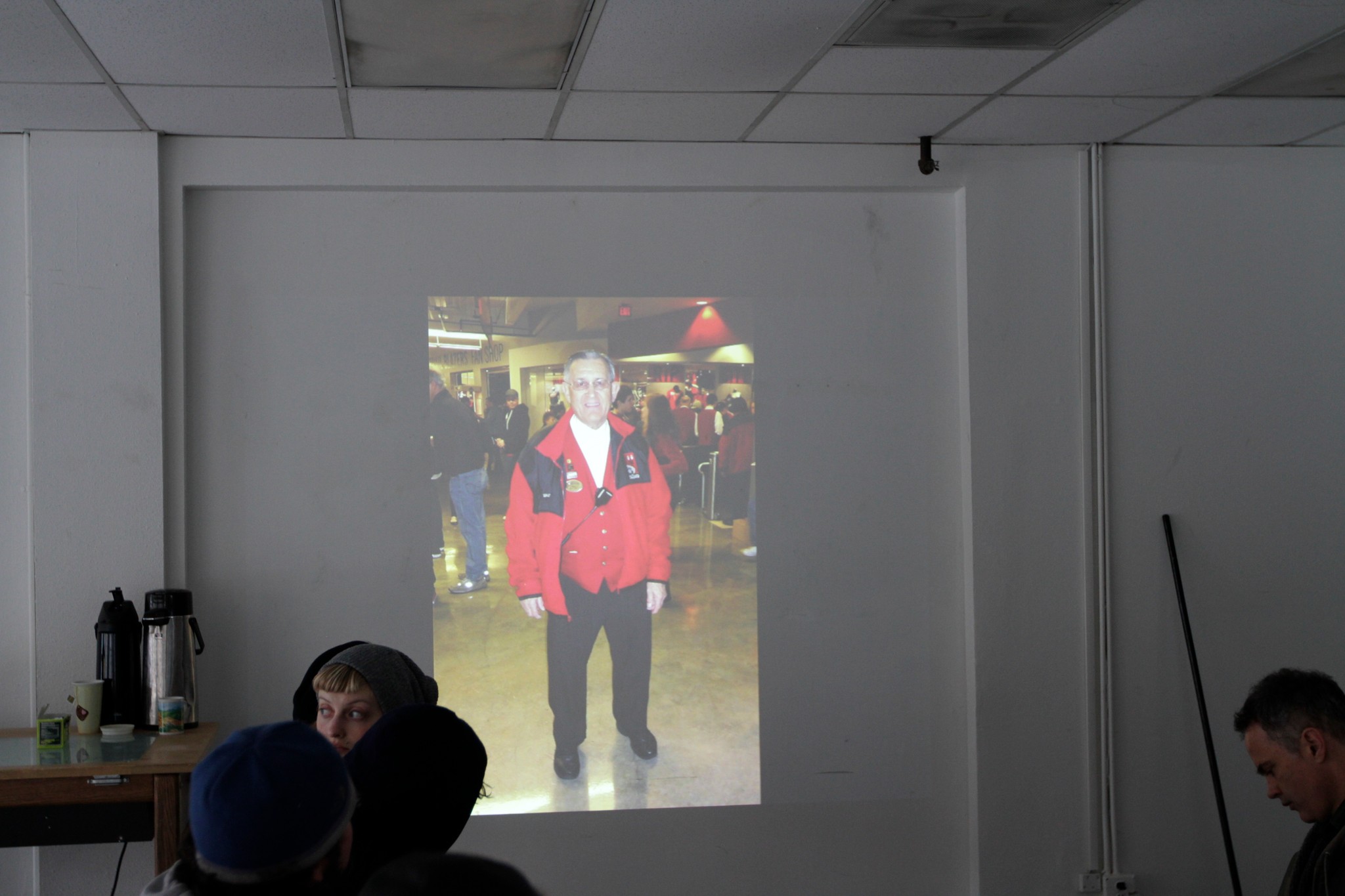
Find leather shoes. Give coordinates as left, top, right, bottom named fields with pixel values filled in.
left=554, top=740, right=581, bottom=780
left=615, top=720, right=657, bottom=760
left=458, top=571, right=491, bottom=581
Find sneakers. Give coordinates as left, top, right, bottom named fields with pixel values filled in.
left=447, top=577, right=489, bottom=594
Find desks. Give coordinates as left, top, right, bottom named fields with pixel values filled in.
left=0, top=725, right=246, bottom=880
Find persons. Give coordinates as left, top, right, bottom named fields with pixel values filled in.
left=502, top=351, right=674, bottom=780
left=288, top=642, right=441, bottom=758
left=1233, top=666, right=1345, bottom=896
left=340, top=700, right=489, bottom=896
left=136, top=719, right=359, bottom=896
left=428, top=368, right=757, bottom=605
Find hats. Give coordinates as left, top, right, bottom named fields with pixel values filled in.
left=187, top=722, right=356, bottom=880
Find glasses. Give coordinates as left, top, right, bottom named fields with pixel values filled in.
left=564, top=377, right=615, bottom=391
